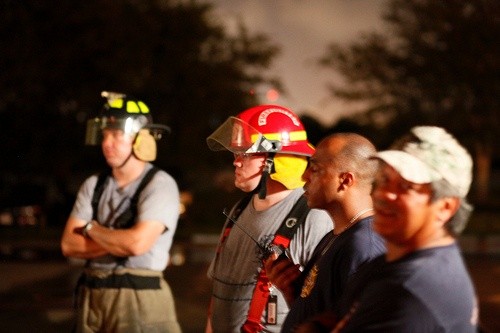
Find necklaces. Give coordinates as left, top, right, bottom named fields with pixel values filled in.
left=321, top=207, right=373, bottom=255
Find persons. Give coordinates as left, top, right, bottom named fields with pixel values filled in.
left=281, top=132, right=387, bottom=333
left=317, top=126, right=478, bottom=333
left=61, top=91, right=182, bottom=333
left=206, top=107, right=333, bottom=333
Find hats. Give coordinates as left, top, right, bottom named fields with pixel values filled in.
left=366, top=126, right=474, bottom=197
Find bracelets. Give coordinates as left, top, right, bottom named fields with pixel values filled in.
left=83, top=220, right=97, bottom=237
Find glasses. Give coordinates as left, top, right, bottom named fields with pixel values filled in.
left=234, top=153, right=266, bottom=161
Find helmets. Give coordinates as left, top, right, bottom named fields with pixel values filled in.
left=84, top=98, right=171, bottom=151
left=206, top=103, right=316, bottom=157
